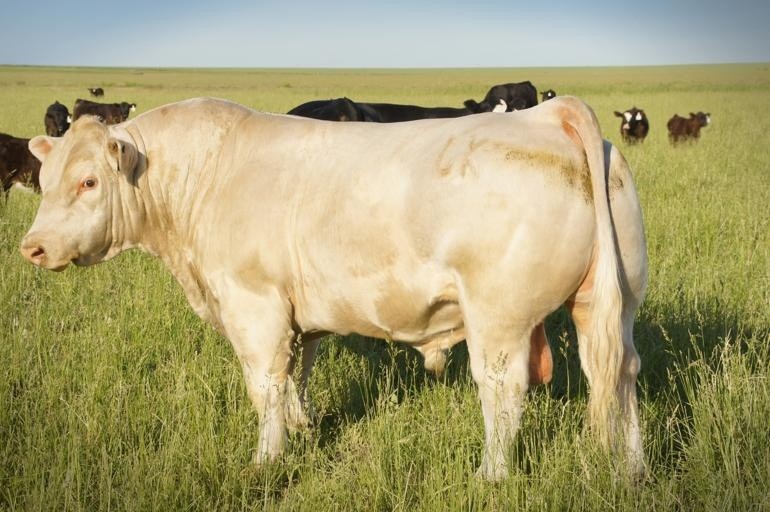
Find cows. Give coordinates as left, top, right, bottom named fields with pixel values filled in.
left=540, top=87, right=556, bottom=101
left=666, top=111, right=711, bottom=147
left=87, top=87, right=105, bottom=98
left=0, top=132, right=42, bottom=205
left=18, top=93, right=649, bottom=487
left=287, top=80, right=539, bottom=124
left=613, top=107, right=649, bottom=145
left=44, top=99, right=72, bottom=137
left=72, top=98, right=136, bottom=127
